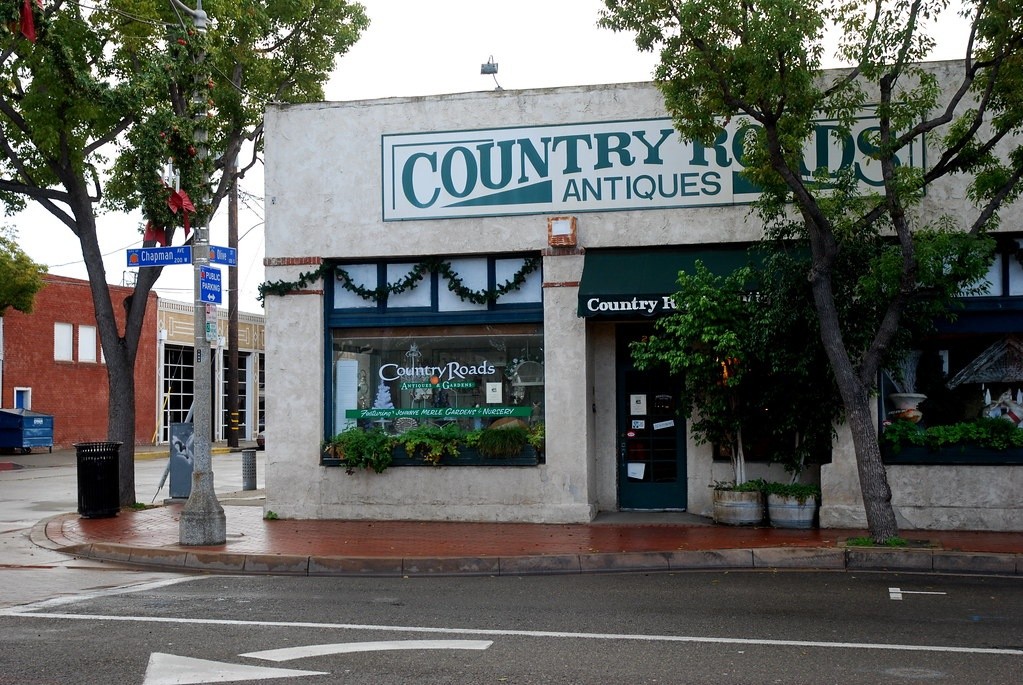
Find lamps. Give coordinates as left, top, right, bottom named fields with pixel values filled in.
left=480, top=55, right=503, bottom=95
left=547, top=216, right=578, bottom=249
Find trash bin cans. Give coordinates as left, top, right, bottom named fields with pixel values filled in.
left=0, top=408, right=54, bottom=454
left=73, top=441, right=124, bottom=519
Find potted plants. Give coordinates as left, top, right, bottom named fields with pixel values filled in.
left=713, top=475, right=766, bottom=525
left=768, top=483, right=820, bottom=531
left=881, top=350, right=929, bottom=421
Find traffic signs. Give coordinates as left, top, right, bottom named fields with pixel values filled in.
left=126, top=245, right=237, bottom=305
